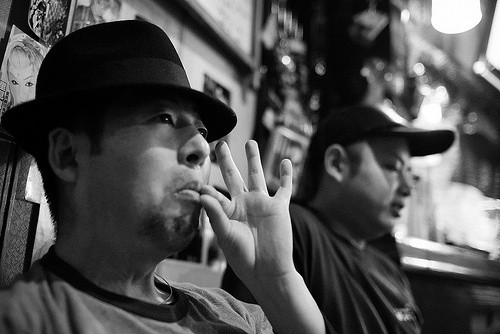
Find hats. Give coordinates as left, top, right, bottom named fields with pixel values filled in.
left=0, top=20, right=237, bottom=142
left=310, top=105, right=455, bottom=156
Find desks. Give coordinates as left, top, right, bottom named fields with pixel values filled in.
left=392, top=234, right=500, bottom=334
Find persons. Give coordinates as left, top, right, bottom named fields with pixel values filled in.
left=218, top=104, right=456, bottom=334
left=0, top=21, right=325, bottom=334
left=5, top=34, right=47, bottom=111
